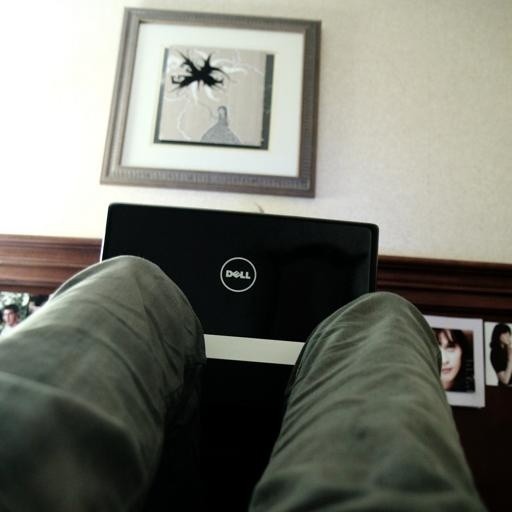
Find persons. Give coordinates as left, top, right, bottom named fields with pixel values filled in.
left=432, top=328, right=468, bottom=392
left=199, top=105, right=241, bottom=144
left=0, top=252, right=489, bottom=512
left=0, top=304, right=21, bottom=336
left=490, top=323, right=512, bottom=388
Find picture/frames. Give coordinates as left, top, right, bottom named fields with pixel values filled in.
left=97, top=8, right=321, bottom=199
left=420, top=314, right=485, bottom=409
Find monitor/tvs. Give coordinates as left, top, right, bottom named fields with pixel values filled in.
left=102, top=202, right=379, bottom=367
